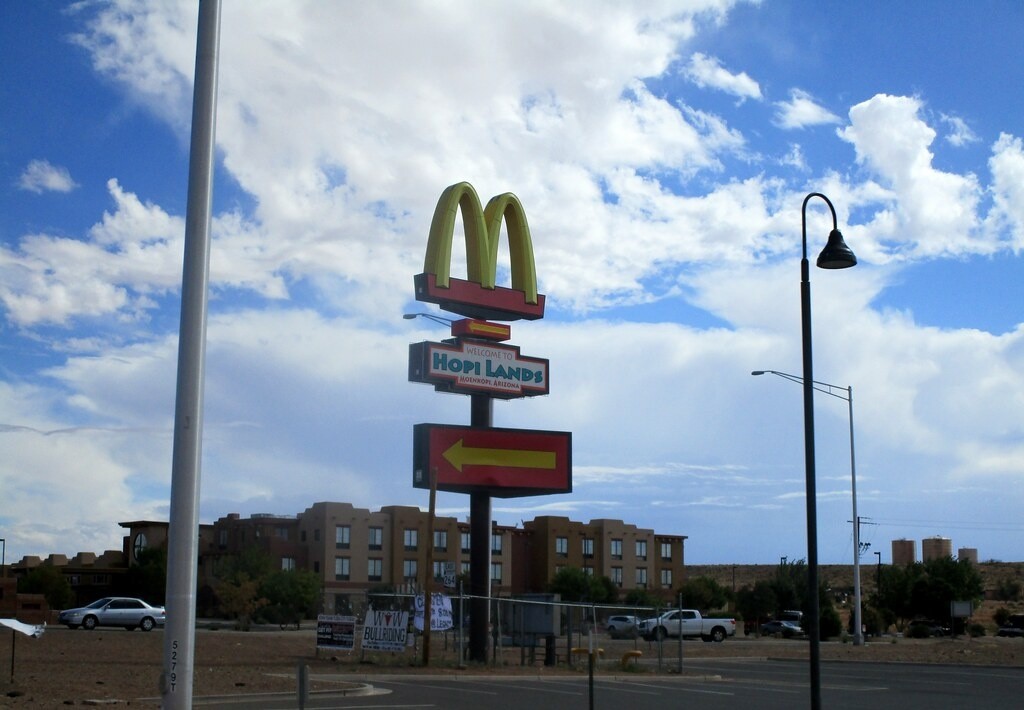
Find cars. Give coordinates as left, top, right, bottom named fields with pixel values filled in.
left=758, top=620, right=804, bottom=640
left=56, top=597, right=167, bottom=631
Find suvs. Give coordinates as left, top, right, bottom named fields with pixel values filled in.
left=608, top=615, right=642, bottom=640
left=905, top=619, right=951, bottom=639
left=998, top=623, right=1024, bottom=638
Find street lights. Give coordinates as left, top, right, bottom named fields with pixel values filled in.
left=874, top=551, right=882, bottom=597
left=1, top=539, right=6, bottom=585
left=799, top=191, right=859, bottom=710
left=751, top=369, right=867, bottom=647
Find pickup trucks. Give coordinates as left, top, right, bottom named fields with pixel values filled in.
left=638, top=609, right=737, bottom=643
